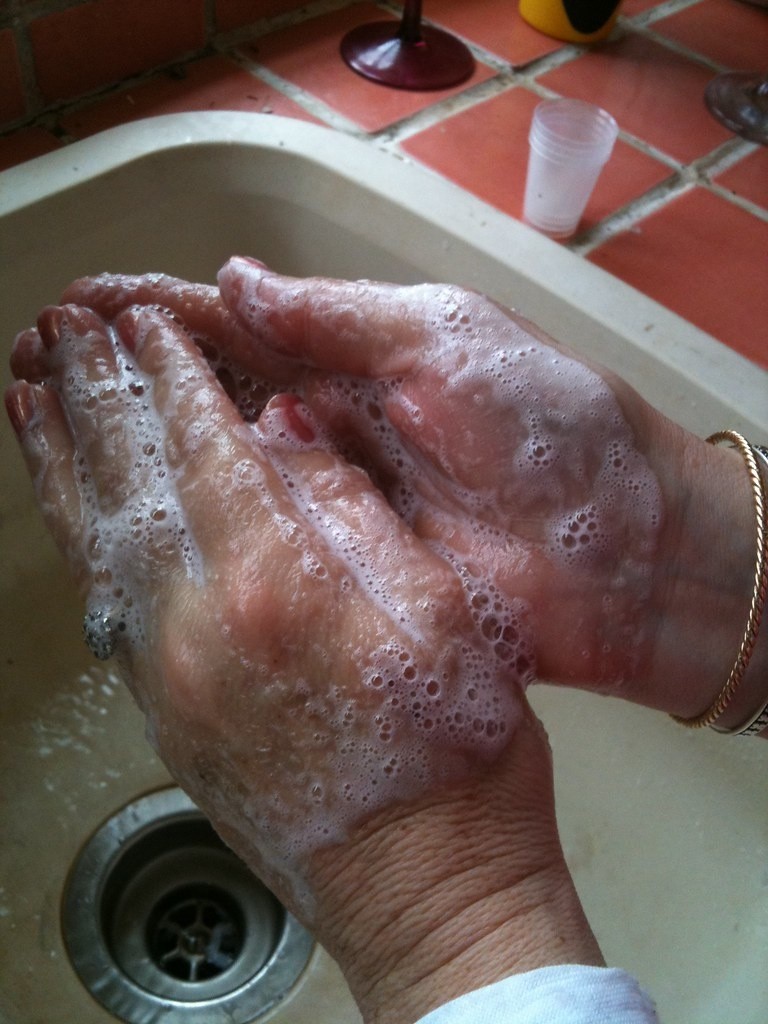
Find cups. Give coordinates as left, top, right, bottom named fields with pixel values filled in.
left=521, top=97, right=618, bottom=240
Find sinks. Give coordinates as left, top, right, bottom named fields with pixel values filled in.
left=0, top=108, right=766, bottom=1023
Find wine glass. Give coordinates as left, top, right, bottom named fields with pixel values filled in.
left=339, top=0, right=475, bottom=92
left=702, top=68, right=768, bottom=146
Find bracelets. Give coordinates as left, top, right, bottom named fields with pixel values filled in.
left=669, top=429, right=768, bottom=738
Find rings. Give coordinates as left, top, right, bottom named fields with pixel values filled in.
left=80, top=603, right=130, bottom=661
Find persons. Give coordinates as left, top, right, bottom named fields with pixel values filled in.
left=0, top=254, right=768, bottom=1024
